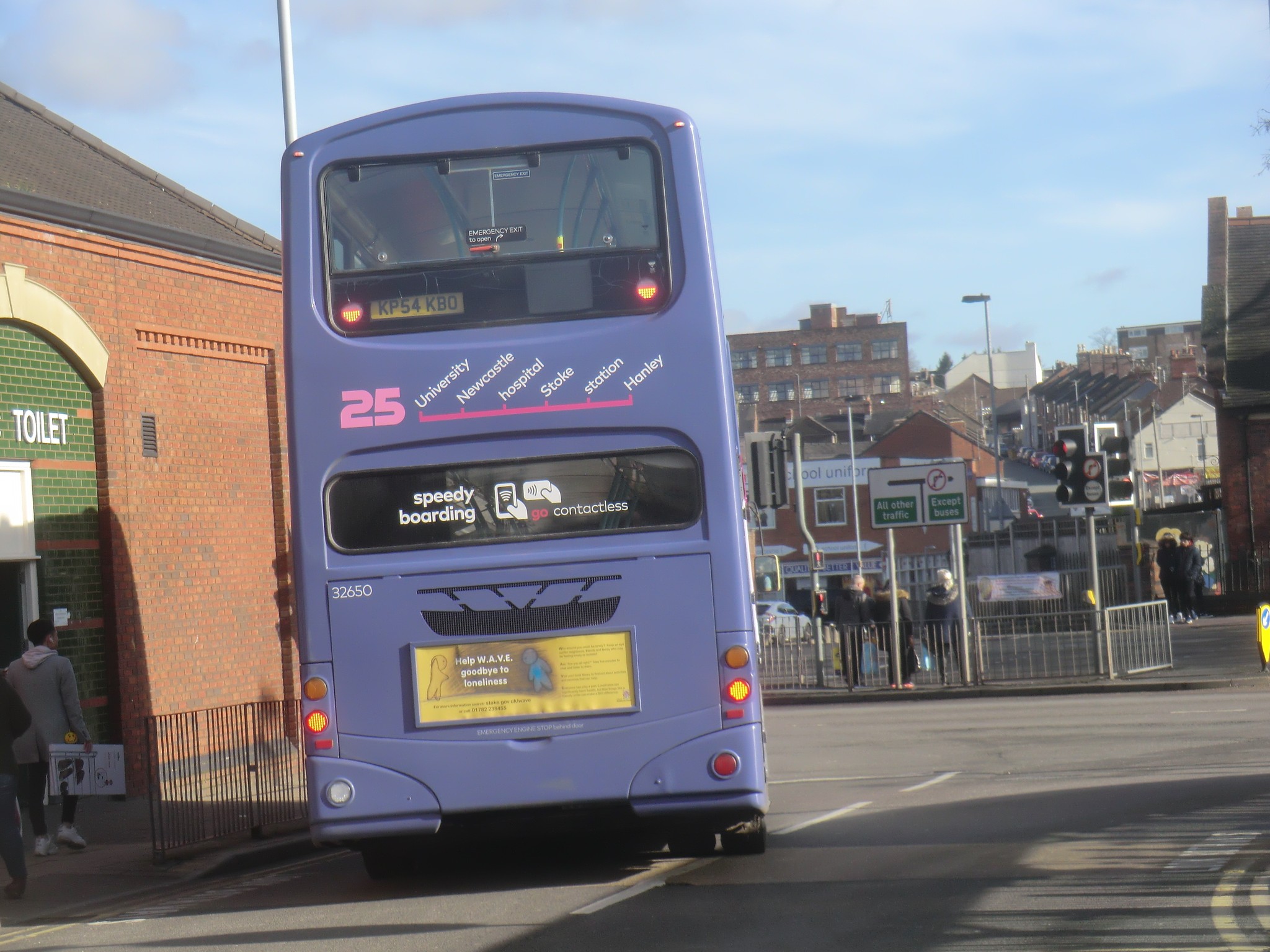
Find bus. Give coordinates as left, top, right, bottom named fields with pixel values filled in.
left=278, top=85, right=779, bottom=879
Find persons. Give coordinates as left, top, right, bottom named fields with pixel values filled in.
left=754, top=565, right=771, bottom=592
left=1242, top=548, right=1262, bottom=600
left=0, top=668, right=33, bottom=897
left=831, top=575, right=869, bottom=691
left=923, top=568, right=978, bottom=684
left=4, top=618, right=93, bottom=857
left=1156, top=531, right=1204, bottom=625
left=872, top=578, right=916, bottom=691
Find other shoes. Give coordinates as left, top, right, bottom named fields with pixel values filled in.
left=1190, top=609, right=1198, bottom=620
left=889, top=683, right=897, bottom=690
left=902, top=682, right=915, bottom=689
left=1185, top=616, right=1192, bottom=624
left=4, top=879, right=26, bottom=897
left=1176, top=612, right=1182, bottom=622
left=1169, top=615, right=1174, bottom=624
left=1199, top=613, right=1214, bottom=618
left=1183, top=616, right=1186, bottom=620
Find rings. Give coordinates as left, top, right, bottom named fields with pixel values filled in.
left=83, top=742, right=87, bottom=744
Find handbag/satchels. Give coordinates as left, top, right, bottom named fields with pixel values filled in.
left=832, top=647, right=849, bottom=680
left=922, top=647, right=938, bottom=672
left=861, top=626, right=879, bottom=676
left=904, top=646, right=921, bottom=674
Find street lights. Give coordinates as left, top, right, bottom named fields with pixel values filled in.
left=1070, top=379, right=1080, bottom=425
left=844, top=393, right=867, bottom=577
left=979, top=395, right=988, bottom=445
left=960, top=292, right=1005, bottom=531
left=1190, top=414, right=1207, bottom=485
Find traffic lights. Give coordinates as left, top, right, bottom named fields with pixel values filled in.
left=812, top=550, right=825, bottom=571
left=813, top=589, right=829, bottom=616
left=1093, top=422, right=1136, bottom=509
left=1049, top=423, right=1110, bottom=509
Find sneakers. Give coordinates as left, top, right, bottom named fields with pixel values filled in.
left=35, top=834, right=59, bottom=856
left=58, top=824, right=87, bottom=850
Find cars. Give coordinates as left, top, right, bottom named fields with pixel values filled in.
left=755, top=600, right=812, bottom=648
left=1016, top=446, right=1056, bottom=475
